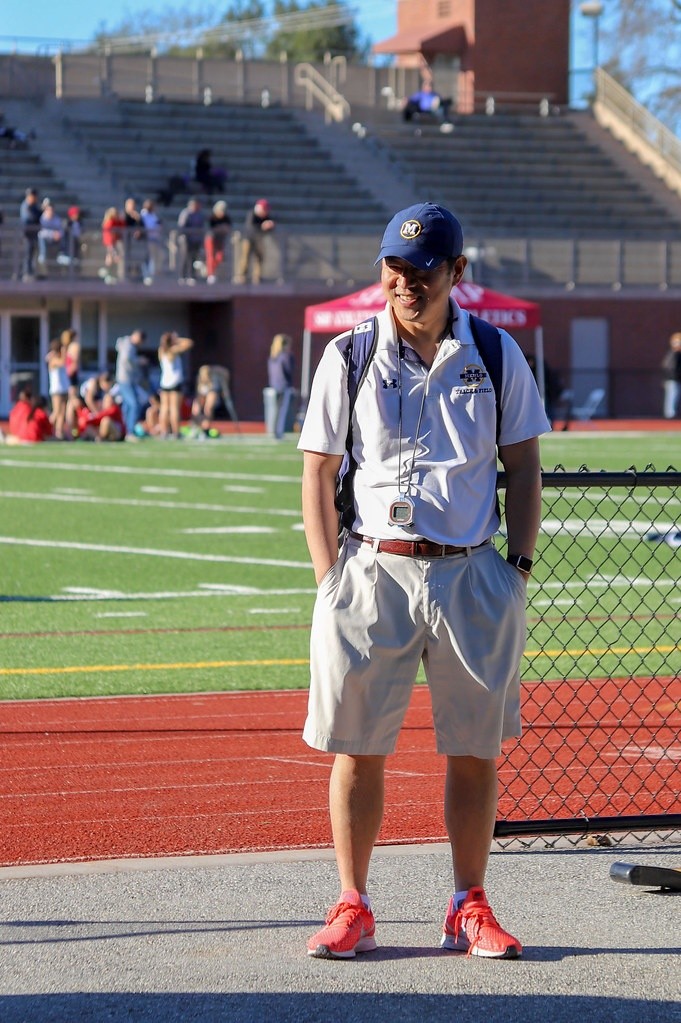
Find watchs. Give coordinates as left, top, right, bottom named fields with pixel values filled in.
left=507, top=554, right=533, bottom=574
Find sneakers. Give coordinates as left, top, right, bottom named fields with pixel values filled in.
left=440, top=886, right=523, bottom=958
left=307, top=888, right=378, bottom=959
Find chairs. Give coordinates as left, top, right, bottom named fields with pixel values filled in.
left=572, top=389, right=605, bottom=420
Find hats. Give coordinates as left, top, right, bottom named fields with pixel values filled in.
left=258, top=198, right=270, bottom=212
left=374, top=202, right=464, bottom=271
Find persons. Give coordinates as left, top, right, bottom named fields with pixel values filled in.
left=21, top=397, right=55, bottom=441
left=73, top=371, right=126, bottom=441
left=140, top=198, right=163, bottom=279
left=158, top=331, right=194, bottom=440
left=115, top=330, right=147, bottom=442
left=524, top=350, right=563, bottom=419
left=142, top=393, right=161, bottom=437
left=36, top=198, right=63, bottom=279
left=176, top=198, right=275, bottom=284
left=267, top=334, right=296, bottom=441
left=9, top=389, right=34, bottom=442
left=195, top=147, right=225, bottom=195
left=20, top=187, right=41, bottom=282
left=61, top=206, right=82, bottom=277
left=191, top=365, right=229, bottom=437
left=46, top=338, right=70, bottom=439
left=403, top=80, right=454, bottom=134
left=123, top=198, right=145, bottom=280
left=660, top=333, right=681, bottom=421
left=97, top=207, right=126, bottom=282
left=59, top=329, right=80, bottom=397
left=296, top=203, right=553, bottom=958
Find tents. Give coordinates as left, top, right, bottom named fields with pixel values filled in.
left=300, top=280, right=545, bottom=411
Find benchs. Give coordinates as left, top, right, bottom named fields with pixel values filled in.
left=0, top=88, right=681, bottom=284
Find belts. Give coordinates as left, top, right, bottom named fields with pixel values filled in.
left=349, top=531, right=491, bottom=560
